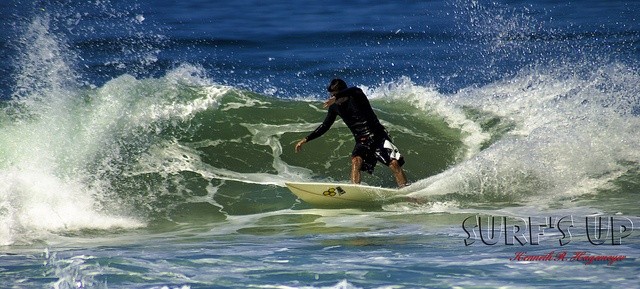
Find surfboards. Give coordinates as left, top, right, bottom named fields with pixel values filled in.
left=284, top=181, right=400, bottom=207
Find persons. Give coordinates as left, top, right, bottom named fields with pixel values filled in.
left=294, top=78, right=409, bottom=187
